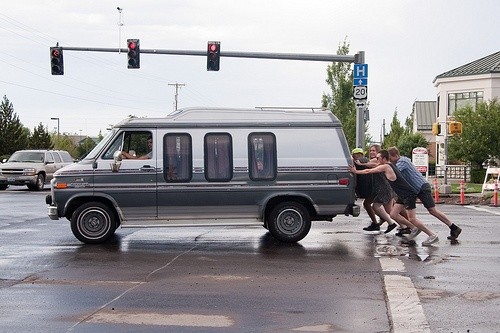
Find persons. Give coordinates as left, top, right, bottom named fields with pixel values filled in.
left=348, top=144, right=463, bottom=246
left=122, top=137, right=153, bottom=159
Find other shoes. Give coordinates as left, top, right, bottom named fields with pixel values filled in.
left=363, top=223, right=380, bottom=231
left=396, top=227, right=411, bottom=236
left=384, top=223, right=397, bottom=233
left=448, top=228, right=462, bottom=240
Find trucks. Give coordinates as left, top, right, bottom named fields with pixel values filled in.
left=45, top=106, right=360, bottom=244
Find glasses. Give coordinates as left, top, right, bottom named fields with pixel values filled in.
left=370, top=150, right=375, bottom=151
left=147, top=141, right=152, bottom=143
left=377, top=157, right=380, bottom=160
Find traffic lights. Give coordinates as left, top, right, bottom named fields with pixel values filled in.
left=127, top=39, right=140, bottom=69
left=206, top=41, right=221, bottom=71
left=50, top=46, right=64, bottom=76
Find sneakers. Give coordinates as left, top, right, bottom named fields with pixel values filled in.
left=410, top=227, right=421, bottom=238
left=422, top=235, right=439, bottom=245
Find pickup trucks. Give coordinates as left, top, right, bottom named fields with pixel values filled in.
left=0, top=150, right=73, bottom=191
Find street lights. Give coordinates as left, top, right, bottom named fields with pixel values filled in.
left=51, top=118, right=59, bottom=147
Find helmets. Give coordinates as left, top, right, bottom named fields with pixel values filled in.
left=352, top=148, right=364, bottom=155
